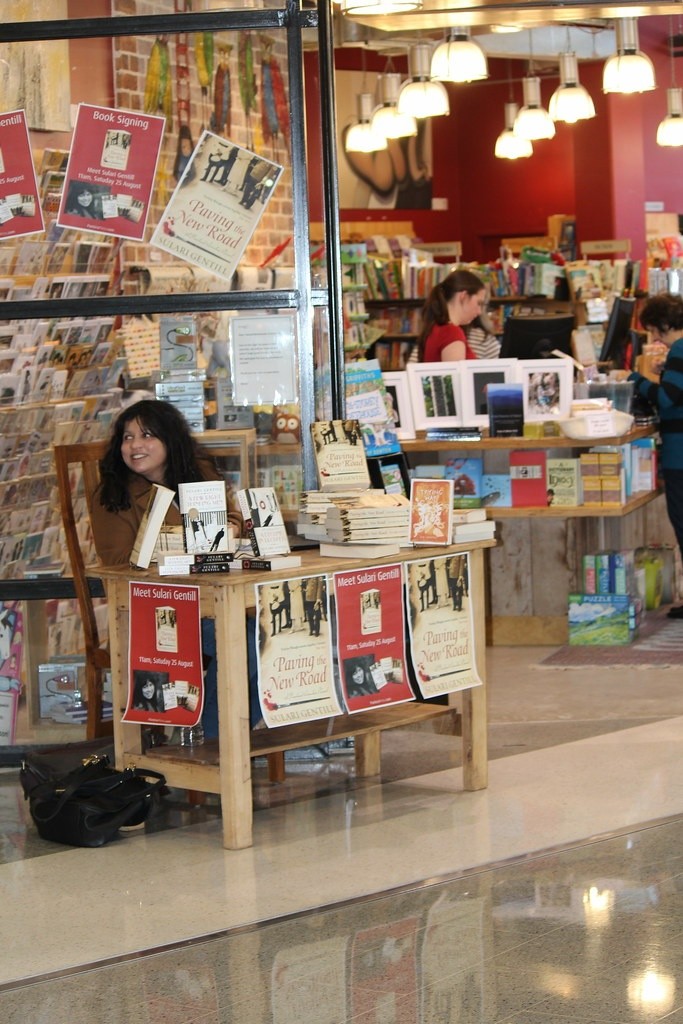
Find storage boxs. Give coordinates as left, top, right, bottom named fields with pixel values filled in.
left=582, top=476, right=601, bottom=506
left=50, top=655, right=89, bottom=702
left=583, top=549, right=601, bottom=594
left=630, top=622, right=641, bottom=643
left=38, top=664, right=78, bottom=719
left=614, top=550, right=636, bottom=596
left=629, top=597, right=643, bottom=617
left=568, top=592, right=631, bottom=647
left=598, top=454, right=622, bottom=479
left=581, top=453, right=599, bottom=477
left=628, top=610, right=643, bottom=630
left=595, top=550, right=618, bottom=593
left=601, top=479, right=622, bottom=507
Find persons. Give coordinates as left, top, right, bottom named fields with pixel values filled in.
left=347, top=658, right=378, bottom=698
left=417, top=269, right=487, bottom=363
left=132, top=671, right=160, bottom=712
left=407, top=313, right=501, bottom=368
left=90, top=402, right=263, bottom=746
left=268, top=576, right=327, bottom=637
left=417, top=554, right=468, bottom=612
left=617, top=292, right=683, bottom=619
left=64, top=181, right=97, bottom=219
left=200, top=139, right=278, bottom=210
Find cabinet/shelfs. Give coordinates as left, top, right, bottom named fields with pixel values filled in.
left=204, top=424, right=683, bottom=648
left=365, top=296, right=557, bottom=373
left=309, top=243, right=371, bottom=363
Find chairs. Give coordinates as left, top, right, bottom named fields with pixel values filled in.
left=53, top=439, right=287, bottom=805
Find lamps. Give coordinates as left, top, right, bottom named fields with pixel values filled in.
left=600, top=16, right=658, bottom=94
left=370, top=55, right=418, bottom=140
left=494, top=58, right=534, bottom=160
left=656, top=15, right=683, bottom=147
left=396, top=41, right=451, bottom=119
left=343, top=0, right=424, bottom=15
left=548, top=25, right=597, bottom=124
left=343, top=44, right=389, bottom=153
left=429, top=26, right=490, bottom=83
left=511, top=27, right=556, bottom=141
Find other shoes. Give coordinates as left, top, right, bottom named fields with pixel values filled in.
left=666, top=605, right=683, bottom=618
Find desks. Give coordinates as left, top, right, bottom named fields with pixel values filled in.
left=83, top=537, right=500, bottom=853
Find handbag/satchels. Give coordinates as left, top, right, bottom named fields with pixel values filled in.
left=29, top=754, right=166, bottom=849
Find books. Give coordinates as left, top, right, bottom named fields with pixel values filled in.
left=217, top=376, right=254, bottom=430
left=157, top=563, right=230, bottom=576
left=296, top=489, right=411, bottom=558
left=129, top=483, right=176, bottom=570
left=197, top=404, right=303, bottom=511
left=159, top=552, right=234, bottom=566
left=310, top=419, right=372, bottom=492
left=0, top=146, right=128, bottom=746
left=568, top=544, right=664, bottom=644
left=227, top=555, right=301, bottom=570
left=360, top=588, right=382, bottom=635
left=237, top=487, right=292, bottom=557
left=155, top=317, right=207, bottom=434
left=411, top=479, right=496, bottom=547
left=309, top=235, right=683, bottom=508
left=178, top=480, right=229, bottom=554
